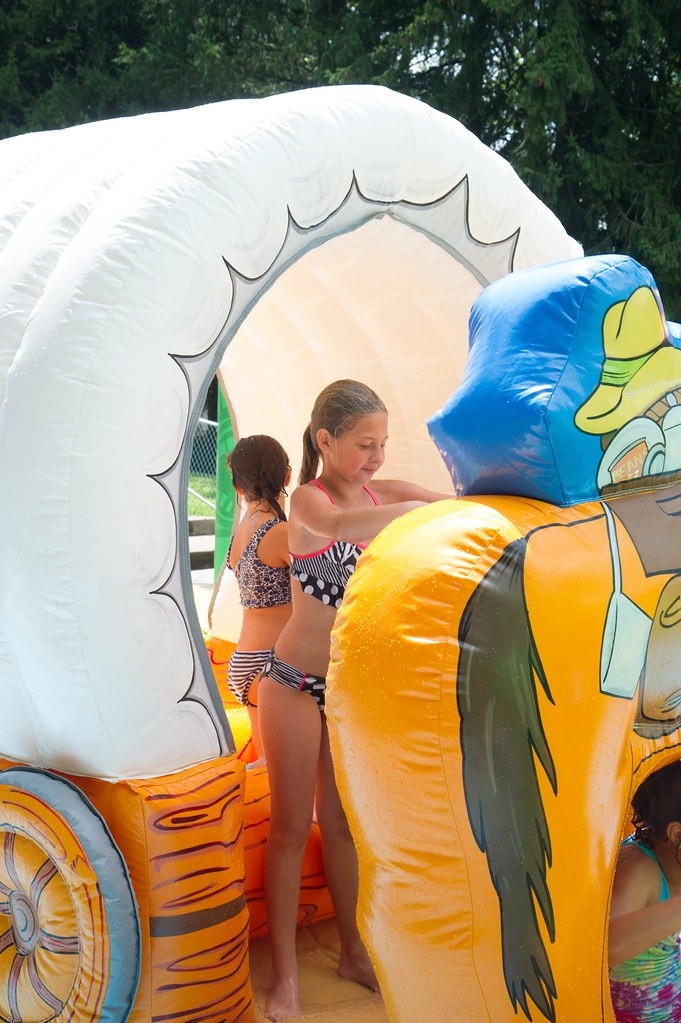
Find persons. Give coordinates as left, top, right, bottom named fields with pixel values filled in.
left=224, top=378, right=458, bottom=1023
left=607, top=756, right=681, bottom=1023
